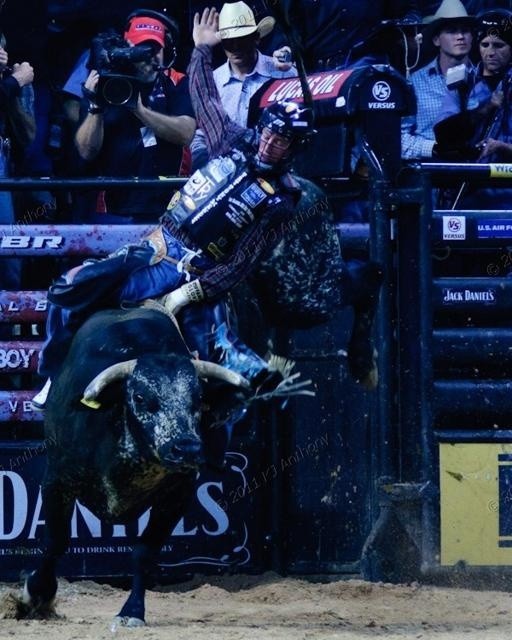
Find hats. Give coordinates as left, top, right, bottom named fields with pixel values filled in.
left=126, top=15, right=166, bottom=53
left=218, top=1, right=276, bottom=41
left=471, top=5, right=512, bottom=39
left=423, top=0, right=480, bottom=29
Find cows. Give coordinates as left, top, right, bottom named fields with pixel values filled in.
left=18, top=300, right=250, bottom=625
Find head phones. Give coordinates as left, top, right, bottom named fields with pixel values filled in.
left=120, top=9, right=178, bottom=54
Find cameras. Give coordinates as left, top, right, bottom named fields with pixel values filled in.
left=80, top=32, right=154, bottom=109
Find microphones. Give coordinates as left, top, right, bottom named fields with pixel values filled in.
left=153, top=60, right=164, bottom=72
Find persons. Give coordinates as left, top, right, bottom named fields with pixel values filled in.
left=72, top=6, right=195, bottom=218
left=188, top=2, right=299, bottom=164
left=401, top=0, right=512, bottom=164
left=0, top=41, right=35, bottom=290
left=476, top=8, right=512, bottom=86
left=35, top=103, right=338, bottom=419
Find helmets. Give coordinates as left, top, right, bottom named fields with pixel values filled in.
left=261, top=105, right=317, bottom=169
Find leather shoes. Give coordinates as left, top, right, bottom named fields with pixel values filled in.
left=32, top=378, right=50, bottom=410
